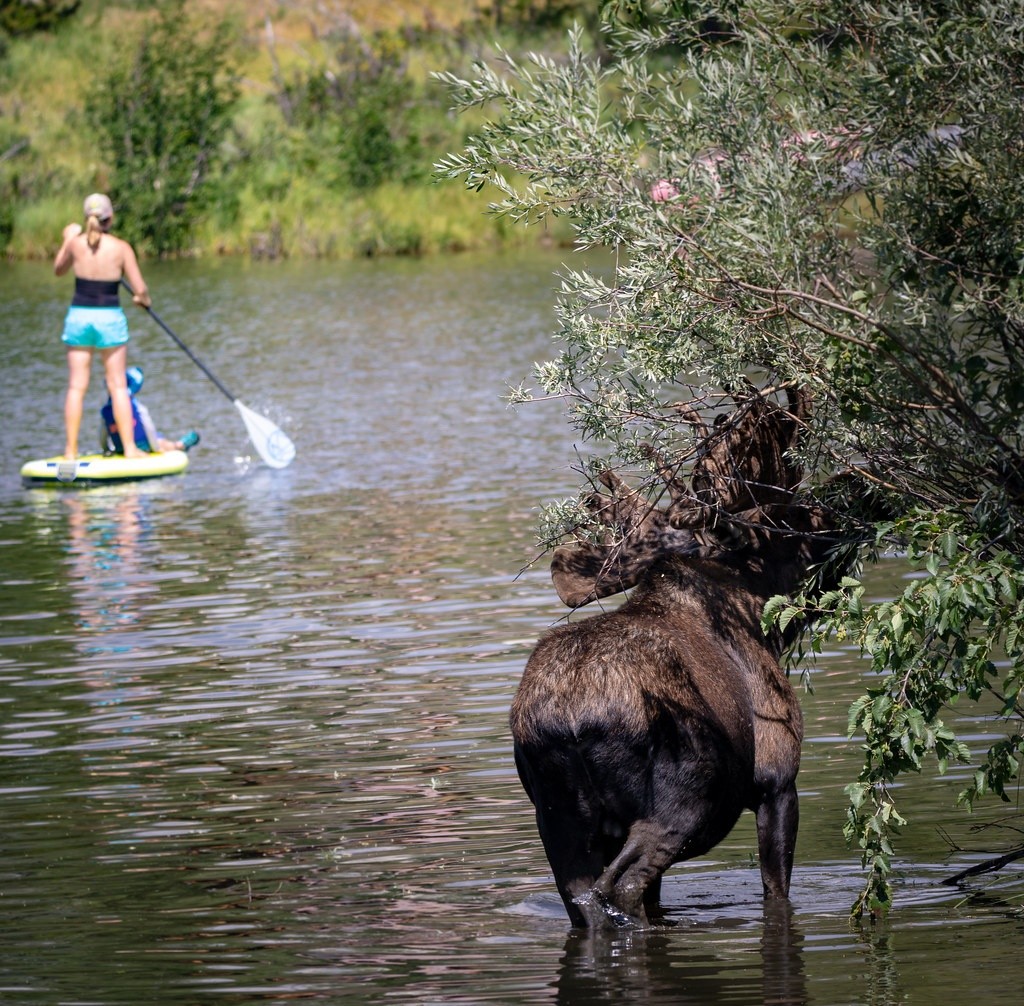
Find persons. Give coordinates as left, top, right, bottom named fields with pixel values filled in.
left=53, top=193, right=152, bottom=460
left=99, top=366, right=199, bottom=456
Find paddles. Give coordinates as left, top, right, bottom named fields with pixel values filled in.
left=120, top=279, right=296, bottom=470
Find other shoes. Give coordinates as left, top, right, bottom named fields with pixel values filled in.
left=182, top=430, right=201, bottom=450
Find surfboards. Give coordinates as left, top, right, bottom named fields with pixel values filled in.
left=20, top=450, right=188, bottom=487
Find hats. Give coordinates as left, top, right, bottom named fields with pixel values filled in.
left=102, top=366, right=144, bottom=397
left=83, top=193, right=114, bottom=222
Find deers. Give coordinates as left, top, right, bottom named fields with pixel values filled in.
left=505, top=379, right=875, bottom=923
